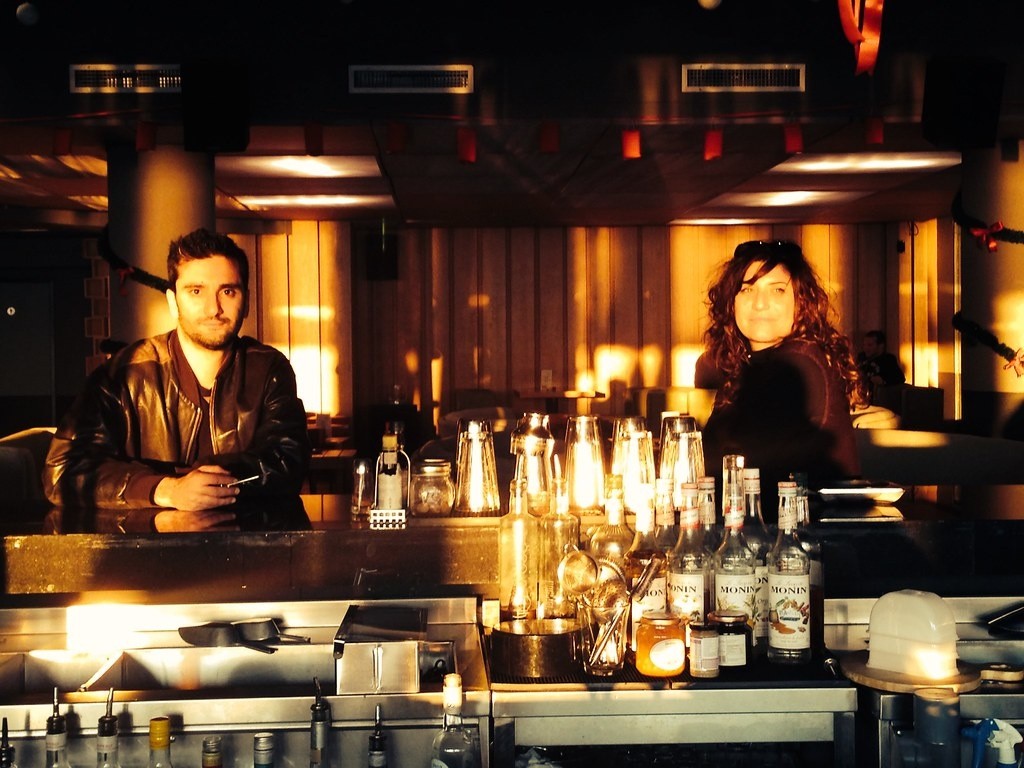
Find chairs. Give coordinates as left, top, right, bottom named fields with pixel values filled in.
left=350, top=391, right=945, bottom=475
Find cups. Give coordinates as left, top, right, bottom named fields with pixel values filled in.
left=577, top=600, right=630, bottom=675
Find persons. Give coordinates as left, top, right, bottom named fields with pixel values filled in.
left=688, top=238, right=908, bottom=484
left=41, top=229, right=311, bottom=513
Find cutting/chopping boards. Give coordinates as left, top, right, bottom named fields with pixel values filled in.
left=841, top=649, right=1024, bottom=693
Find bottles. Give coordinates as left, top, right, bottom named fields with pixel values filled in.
left=351, top=418, right=409, bottom=513
left=538, top=479, right=579, bottom=619
left=673, top=483, right=715, bottom=655
left=376, top=435, right=402, bottom=511
left=411, top=458, right=454, bottom=517
left=431, top=674, right=479, bottom=768
left=623, top=484, right=667, bottom=666
left=0, top=716, right=17, bottom=768
left=147, top=717, right=275, bottom=768
left=46, top=687, right=71, bottom=768
left=591, top=475, right=635, bottom=559
left=456, top=418, right=500, bottom=514
left=767, top=472, right=822, bottom=654
left=498, top=480, right=536, bottom=619
left=96, top=687, right=122, bottom=768
left=511, top=413, right=607, bottom=517
left=714, top=455, right=774, bottom=648
left=611, top=416, right=705, bottom=514
left=698, top=476, right=723, bottom=554
left=655, top=478, right=675, bottom=556
left=310, top=677, right=388, bottom=768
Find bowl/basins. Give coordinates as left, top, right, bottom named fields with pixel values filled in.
left=490, top=619, right=579, bottom=678
left=866, top=590, right=961, bottom=679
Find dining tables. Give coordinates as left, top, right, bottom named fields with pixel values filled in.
left=513, top=392, right=605, bottom=414
